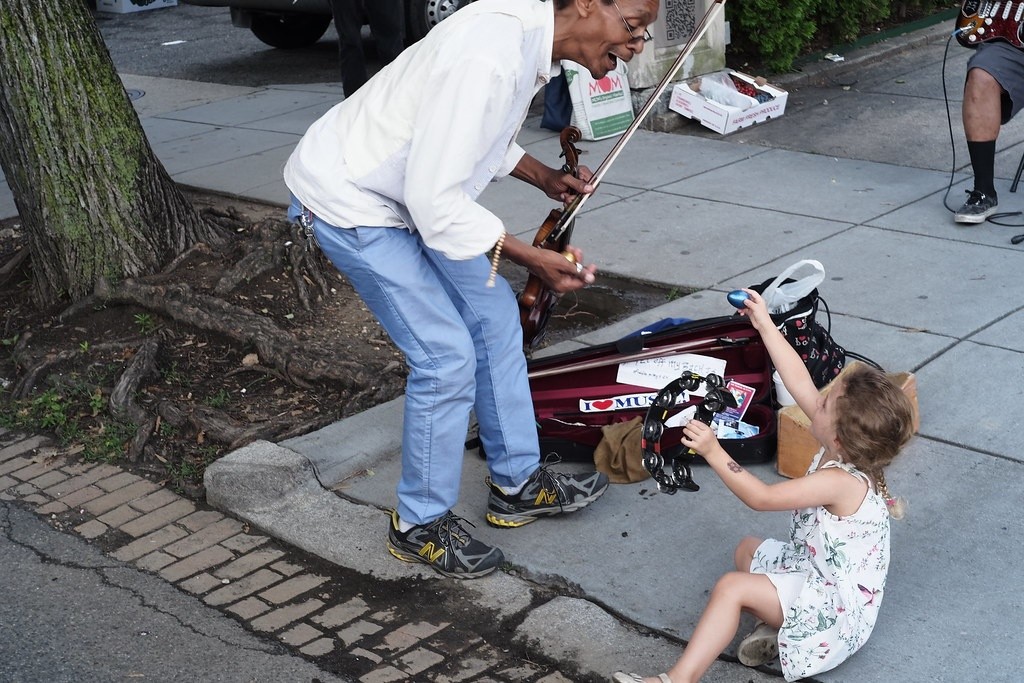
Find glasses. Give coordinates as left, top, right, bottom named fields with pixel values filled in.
left=613, top=0, right=655, bottom=45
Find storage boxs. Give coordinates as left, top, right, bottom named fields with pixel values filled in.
left=669, top=70, right=788, bottom=135
left=96, top=0, right=177, bottom=14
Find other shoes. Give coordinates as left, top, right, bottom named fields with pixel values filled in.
left=737, top=622, right=779, bottom=666
left=612, top=671, right=672, bottom=683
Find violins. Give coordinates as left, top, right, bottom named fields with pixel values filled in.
left=516, top=125, right=585, bottom=345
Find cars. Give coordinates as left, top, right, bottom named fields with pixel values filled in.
left=175, top=0, right=468, bottom=54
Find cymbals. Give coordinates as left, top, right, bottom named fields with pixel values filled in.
left=639, top=370, right=737, bottom=496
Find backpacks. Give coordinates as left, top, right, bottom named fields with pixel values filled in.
left=732, top=275, right=846, bottom=391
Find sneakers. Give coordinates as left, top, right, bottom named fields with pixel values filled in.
left=387, top=509, right=505, bottom=579
left=955, top=187, right=998, bottom=223
left=485, top=451, right=610, bottom=530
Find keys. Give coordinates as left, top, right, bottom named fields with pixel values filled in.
left=291, top=221, right=319, bottom=253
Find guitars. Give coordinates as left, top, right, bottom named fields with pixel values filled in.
left=954, top=0, right=1024, bottom=50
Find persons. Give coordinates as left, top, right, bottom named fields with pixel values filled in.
left=612, top=287, right=914, bottom=683
left=285, top=0, right=662, bottom=579
left=955, top=40, right=1024, bottom=223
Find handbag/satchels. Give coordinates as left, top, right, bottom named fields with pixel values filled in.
left=561, top=56, right=635, bottom=140
left=540, top=65, right=573, bottom=134
